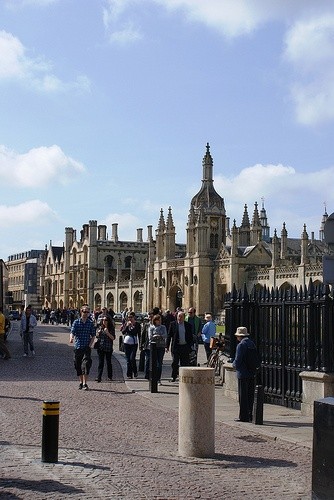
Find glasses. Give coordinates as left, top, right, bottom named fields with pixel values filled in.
left=83, top=311, right=90, bottom=313
left=101, top=319, right=107, bottom=321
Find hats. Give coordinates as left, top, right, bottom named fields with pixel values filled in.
left=234, top=327, right=250, bottom=336
left=204, top=314, right=212, bottom=321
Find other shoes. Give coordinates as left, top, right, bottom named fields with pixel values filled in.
left=78, top=384, right=82, bottom=389
left=83, top=384, right=88, bottom=389
left=31, top=350, right=35, bottom=356
left=23, top=353, right=27, bottom=356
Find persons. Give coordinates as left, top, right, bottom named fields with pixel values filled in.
left=148, top=314, right=167, bottom=385
left=0, top=304, right=217, bottom=380
left=122, top=312, right=141, bottom=379
left=202, top=314, right=215, bottom=365
left=95, top=315, right=116, bottom=383
left=231, top=327, right=260, bottom=422
left=70, top=306, right=96, bottom=390
left=165, top=312, right=193, bottom=382
left=20, top=308, right=37, bottom=357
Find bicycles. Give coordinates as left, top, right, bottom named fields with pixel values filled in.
left=207, top=333, right=230, bottom=376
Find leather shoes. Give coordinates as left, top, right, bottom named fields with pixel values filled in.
left=234, top=418, right=252, bottom=422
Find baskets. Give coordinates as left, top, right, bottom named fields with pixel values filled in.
left=210, top=336, right=224, bottom=348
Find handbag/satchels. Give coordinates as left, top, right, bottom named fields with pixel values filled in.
left=89, top=336, right=101, bottom=349
left=156, top=333, right=167, bottom=348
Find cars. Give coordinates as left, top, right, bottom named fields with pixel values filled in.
left=112, top=312, right=149, bottom=322
